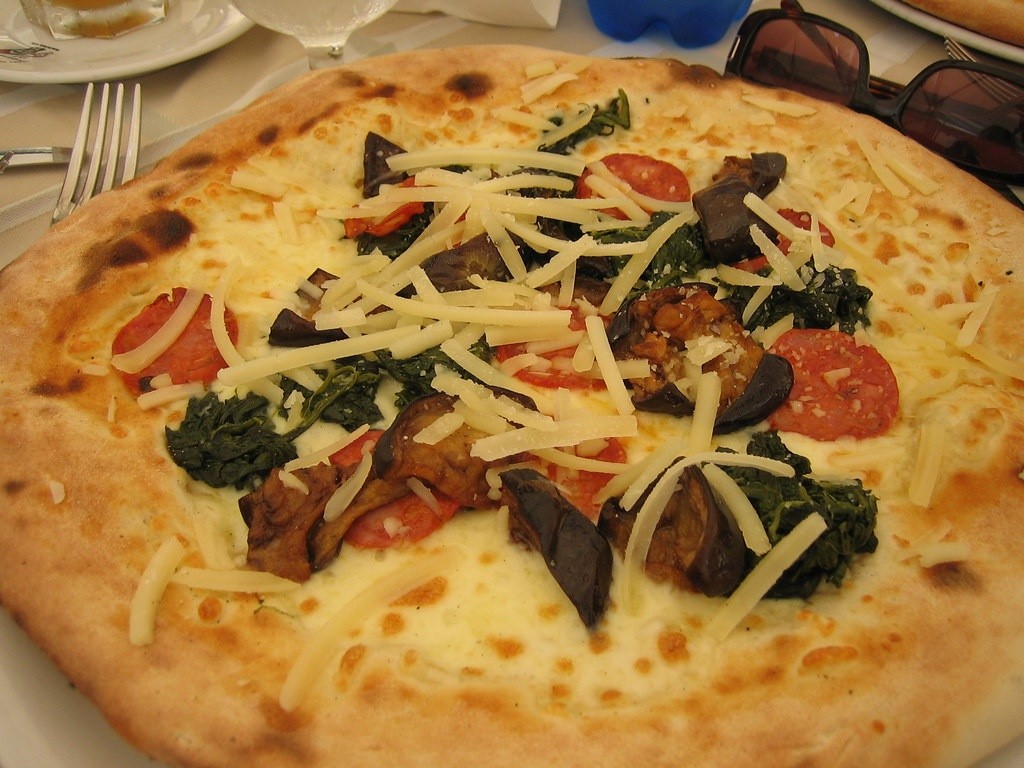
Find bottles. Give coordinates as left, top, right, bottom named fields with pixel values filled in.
left=584, top=0, right=754, bottom=49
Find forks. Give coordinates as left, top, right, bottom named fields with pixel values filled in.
left=51, top=82, right=144, bottom=228
left=943, top=33, right=1024, bottom=113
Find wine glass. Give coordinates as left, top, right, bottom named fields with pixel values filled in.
left=228, top=0, right=400, bottom=73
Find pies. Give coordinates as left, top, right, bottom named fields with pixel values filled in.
left=898, top=0, right=1024, bottom=48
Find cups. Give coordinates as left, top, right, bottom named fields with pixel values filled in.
left=19, top=1, right=170, bottom=41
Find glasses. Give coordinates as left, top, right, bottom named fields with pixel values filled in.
left=723, top=0, right=1024, bottom=212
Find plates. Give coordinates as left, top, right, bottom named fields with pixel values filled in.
left=871, top=0, right=1024, bottom=65
left=0, top=1, right=257, bottom=84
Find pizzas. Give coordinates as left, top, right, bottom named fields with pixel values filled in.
left=0, top=45, right=1024, bottom=768
